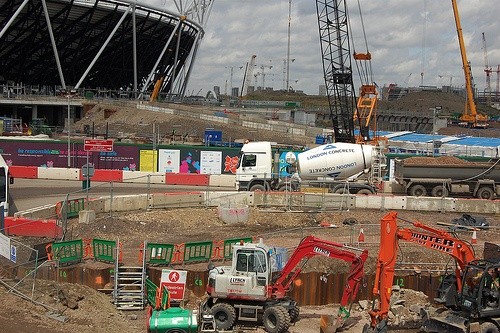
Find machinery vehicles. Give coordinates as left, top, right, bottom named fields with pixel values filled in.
left=362, top=210, right=500, bottom=333
left=204, top=236, right=369, bottom=333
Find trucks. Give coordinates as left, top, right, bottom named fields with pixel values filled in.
left=234, top=141, right=377, bottom=195
left=0, top=154, right=14, bottom=216
left=394, top=156, right=500, bottom=200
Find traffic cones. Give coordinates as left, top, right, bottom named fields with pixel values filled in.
left=471, top=223, right=477, bottom=244
left=359, top=224, right=365, bottom=242
